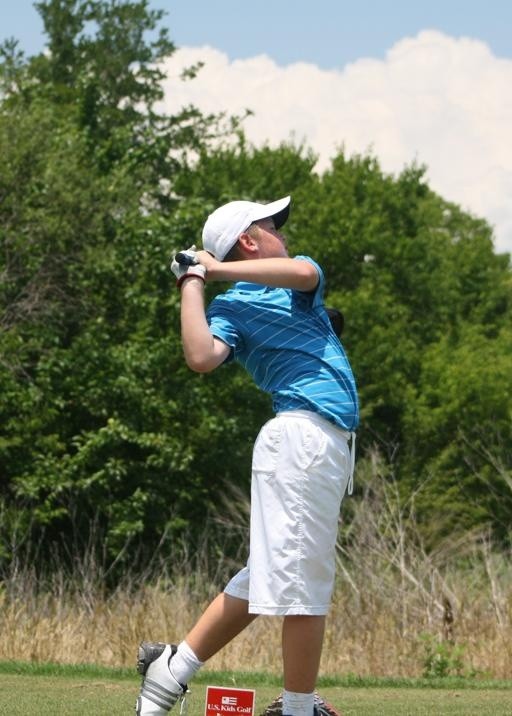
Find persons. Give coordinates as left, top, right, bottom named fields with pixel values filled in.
left=133, top=189, right=361, bottom=715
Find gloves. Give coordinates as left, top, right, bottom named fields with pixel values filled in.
left=170, top=245, right=209, bottom=284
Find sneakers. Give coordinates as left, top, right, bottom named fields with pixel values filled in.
left=135, top=640, right=192, bottom=716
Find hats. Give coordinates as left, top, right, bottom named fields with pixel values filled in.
left=202, top=195, right=291, bottom=262
